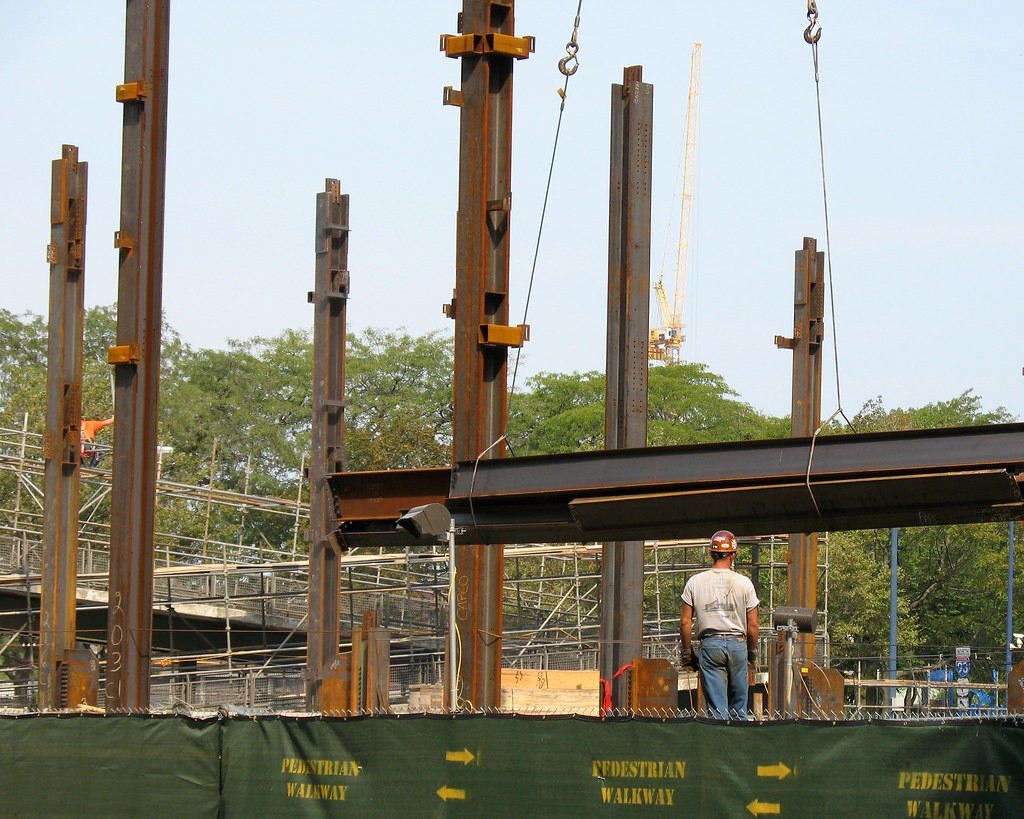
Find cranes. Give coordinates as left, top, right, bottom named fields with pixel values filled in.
left=647, top=41, right=702, bottom=364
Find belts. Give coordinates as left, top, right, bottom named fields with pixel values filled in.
left=703, top=635, right=747, bottom=638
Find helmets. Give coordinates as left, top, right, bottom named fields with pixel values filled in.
left=708, top=530, right=740, bottom=552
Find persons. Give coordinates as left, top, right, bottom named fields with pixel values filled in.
left=680, top=530, right=759, bottom=720
left=81, top=415, right=114, bottom=468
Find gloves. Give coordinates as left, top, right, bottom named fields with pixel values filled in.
left=680, top=645, right=699, bottom=673
left=748, top=646, right=759, bottom=667
